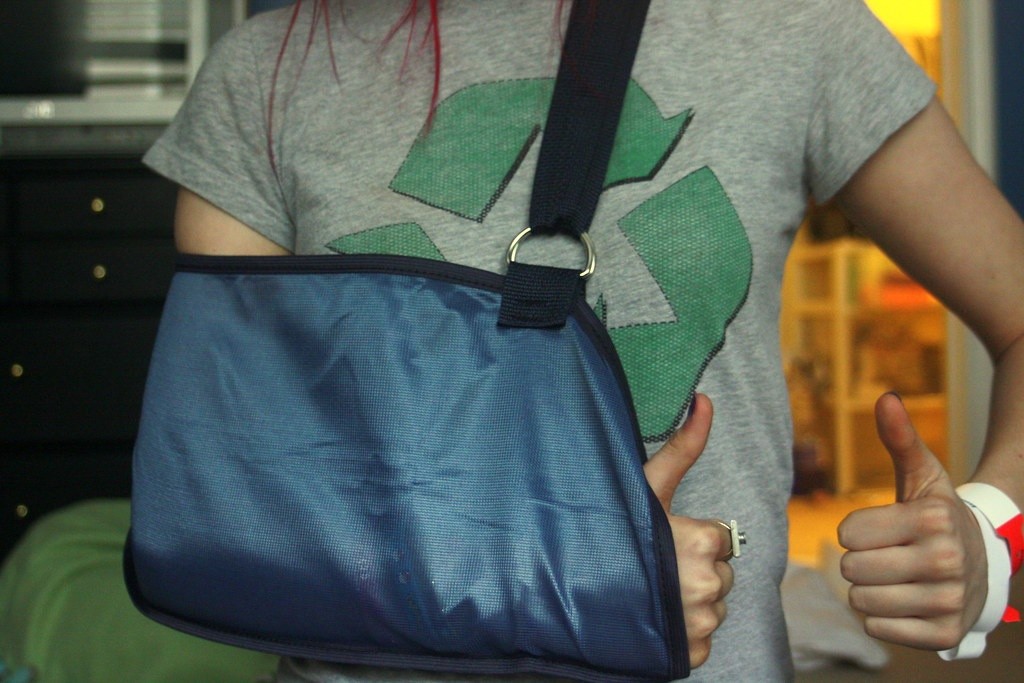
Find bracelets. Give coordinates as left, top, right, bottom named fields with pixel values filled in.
left=936, top=482, right=1024, bottom=660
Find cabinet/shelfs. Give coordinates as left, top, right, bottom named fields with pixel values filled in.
left=0, top=154, right=181, bottom=569
left=791, top=37, right=955, bottom=498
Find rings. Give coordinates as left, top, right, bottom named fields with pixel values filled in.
left=717, top=519, right=746, bottom=560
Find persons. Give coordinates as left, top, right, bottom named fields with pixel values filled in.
left=142, top=0, right=1024, bottom=683
